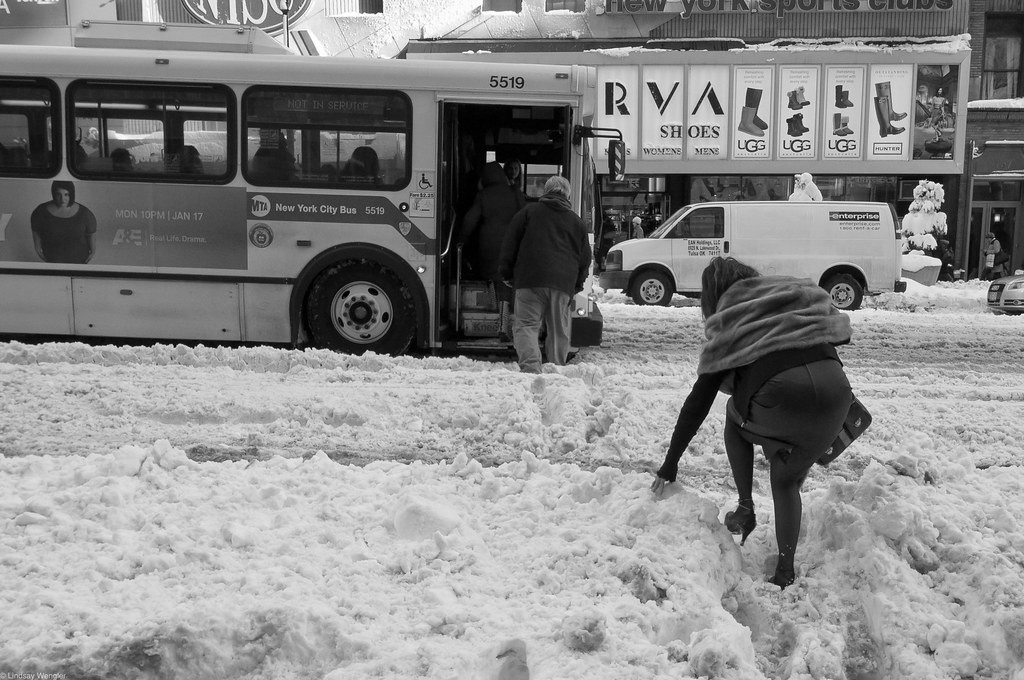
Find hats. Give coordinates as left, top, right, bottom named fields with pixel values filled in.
left=632, top=217, right=641, bottom=224
left=985, top=232, right=994, bottom=238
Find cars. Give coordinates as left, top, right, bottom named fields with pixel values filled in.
left=987, top=273, right=1024, bottom=315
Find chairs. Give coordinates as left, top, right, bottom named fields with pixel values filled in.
left=109, top=149, right=135, bottom=179
left=165, top=144, right=204, bottom=175
left=343, top=160, right=374, bottom=184
left=251, top=149, right=288, bottom=181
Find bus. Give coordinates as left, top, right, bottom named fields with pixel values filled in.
left=0, top=43, right=605, bottom=358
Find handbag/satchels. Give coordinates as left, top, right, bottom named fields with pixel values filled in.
left=815, top=390, right=872, bottom=466
left=986, top=253, right=995, bottom=268
left=991, top=239, right=1008, bottom=265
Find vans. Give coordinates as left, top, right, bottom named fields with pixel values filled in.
left=597, top=200, right=908, bottom=313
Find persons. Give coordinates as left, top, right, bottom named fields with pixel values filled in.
left=929, top=87, right=946, bottom=142
left=110, top=147, right=140, bottom=179
left=632, top=216, right=645, bottom=239
left=172, top=144, right=202, bottom=176
left=338, top=146, right=380, bottom=189
left=788, top=172, right=823, bottom=202
left=454, top=132, right=593, bottom=372
left=251, top=126, right=298, bottom=184
left=982, top=231, right=1003, bottom=281
left=30, top=180, right=100, bottom=265
left=650, top=256, right=850, bottom=592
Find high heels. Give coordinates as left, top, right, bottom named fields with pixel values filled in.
left=724, top=511, right=757, bottom=546
left=764, top=564, right=795, bottom=591
